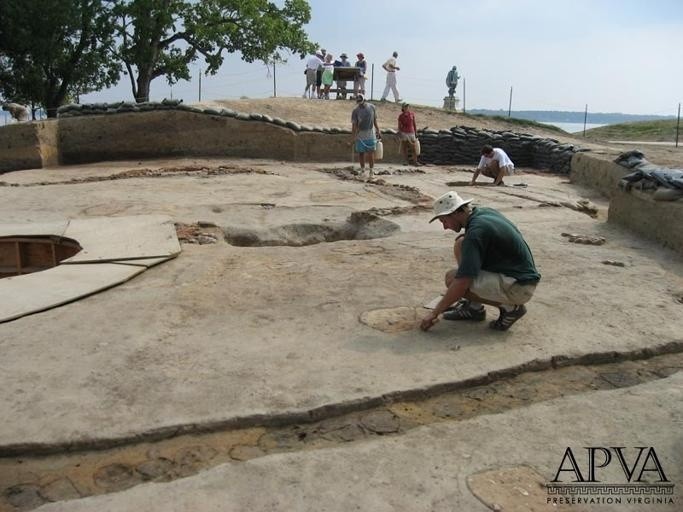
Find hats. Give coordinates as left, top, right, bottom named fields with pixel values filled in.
left=355, top=93, right=365, bottom=104
left=402, top=101, right=409, bottom=107
left=315, top=48, right=364, bottom=60
left=428, top=191, right=474, bottom=224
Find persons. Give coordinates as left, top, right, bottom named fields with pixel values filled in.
left=381, top=51, right=403, bottom=102
left=398, top=102, right=423, bottom=166
left=445, top=65, right=461, bottom=94
left=351, top=94, right=381, bottom=177
left=302, top=48, right=366, bottom=100
left=469, top=144, right=514, bottom=186
left=420, top=191, right=541, bottom=332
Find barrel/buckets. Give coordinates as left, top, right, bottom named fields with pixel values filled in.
left=374, top=139, right=384, bottom=160
left=415, top=138, right=421, bottom=156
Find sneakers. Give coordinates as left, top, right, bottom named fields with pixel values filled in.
left=488, top=304, right=527, bottom=330
left=415, top=162, right=422, bottom=166
left=368, top=172, right=375, bottom=178
left=320, top=93, right=326, bottom=99
left=358, top=172, right=366, bottom=178
left=442, top=300, right=486, bottom=321
